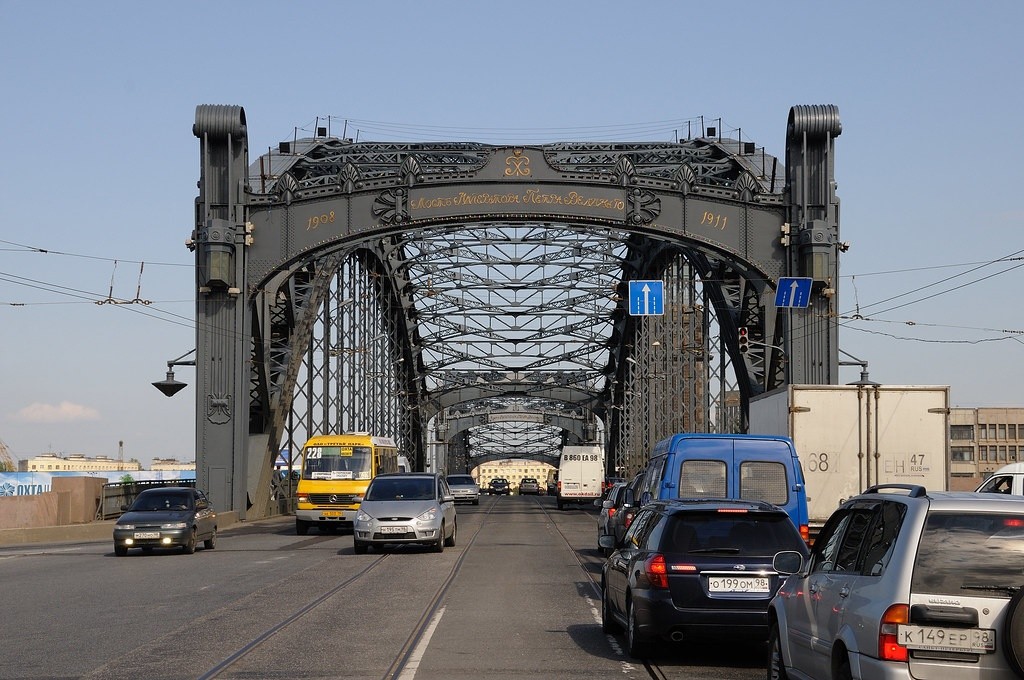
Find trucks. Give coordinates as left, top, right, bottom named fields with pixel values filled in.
left=554, top=444, right=605, bottom=509
left=740, top=382, right=950, bottom=546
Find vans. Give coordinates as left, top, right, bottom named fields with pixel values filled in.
left=635, top=432, right=811, bottom=558
left=939, top=461, right=1024, bottom=494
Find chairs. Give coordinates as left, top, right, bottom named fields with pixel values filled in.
left=494, top=481, right=498, bottom=484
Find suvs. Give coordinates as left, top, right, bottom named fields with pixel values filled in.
left=765, top=484, right=1023, bottom=680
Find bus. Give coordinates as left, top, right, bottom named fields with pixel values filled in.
left=292, top=433, right=400, bottom=535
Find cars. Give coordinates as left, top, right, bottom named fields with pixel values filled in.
left=593, top=472, right=650, bottom=558
left=446, top=474, right=480, bottom=506
left=397, top=456, right=410, bottom=472
left=596, top=497, right=813, bottom=660
left=111, top=485, right=219, bottom=554
left=352, top=471, right=458, bottom=553
left=518, top=478, right=539, bottom=496
left=488, top=478, right=511, bottom=496
